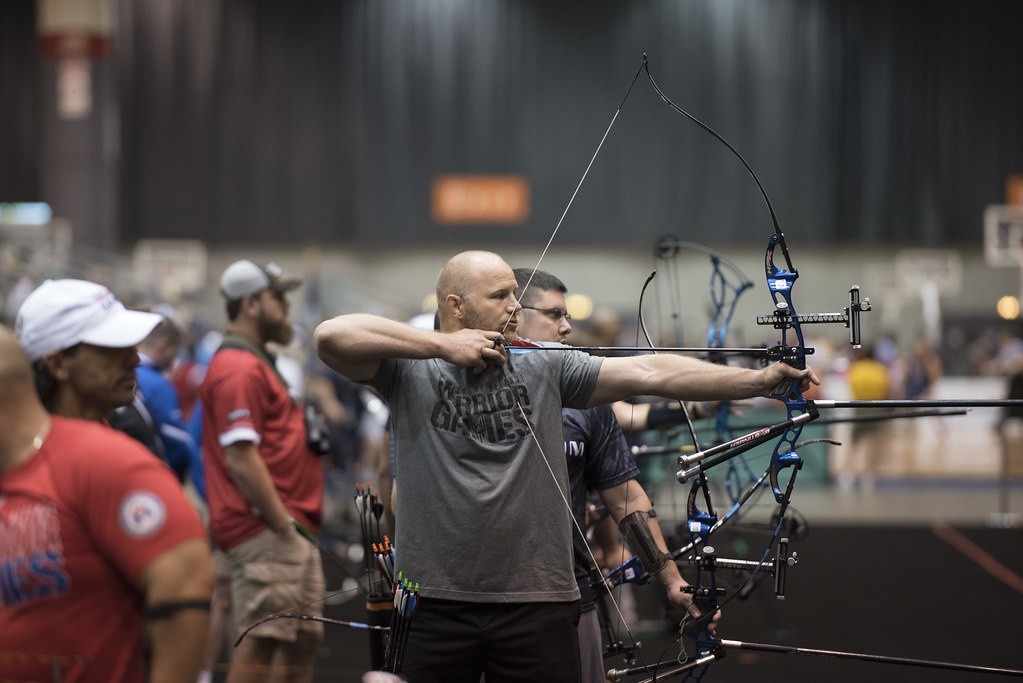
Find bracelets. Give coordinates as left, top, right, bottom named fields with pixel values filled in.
left=274, top=517, right=295, bottom=533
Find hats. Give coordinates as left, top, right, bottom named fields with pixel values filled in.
left=14, top=279, right=164, bottom=362
left=219, top=259, right=302, bottom=301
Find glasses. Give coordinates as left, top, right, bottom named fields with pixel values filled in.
left=523, top=307, right=572, bottom=322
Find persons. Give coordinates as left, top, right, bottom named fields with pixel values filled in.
left=17, top=276, right=165, bottom=428
left=314, top=250, right=821, bottom=683
left=378, top=396, right=754, bottom=639
left=790, top=328, right=1022, bottom=497
left=195, top=257, right=326, bottom=683
left=114, top=299, right=387, bottom=683
left=512, top=268, right=721, bottom=683
left=0, top=324, right=213, bottom=683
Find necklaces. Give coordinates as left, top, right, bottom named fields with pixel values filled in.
left=14, top=421, right=51, bottom=463
